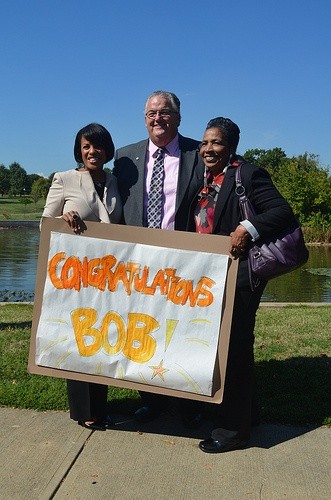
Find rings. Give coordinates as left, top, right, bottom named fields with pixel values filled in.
left=73, top=215, right=78, bottom=219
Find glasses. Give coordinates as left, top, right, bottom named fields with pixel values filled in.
left=146, top=110, right=171, bottom=118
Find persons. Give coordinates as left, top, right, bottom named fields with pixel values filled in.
left=40, top=124, right=123, bottom=432
left=112, top=91, right=206, bottom=424
left=186, top=117, right=299, bottom=454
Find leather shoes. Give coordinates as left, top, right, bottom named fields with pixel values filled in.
left=199, top=437, right=242, bottom=453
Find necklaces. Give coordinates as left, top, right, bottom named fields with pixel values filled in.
left=93, top=179, right=106, bottom=189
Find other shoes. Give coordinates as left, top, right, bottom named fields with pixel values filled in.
left=78, top=419, right=106, bottom=431
left=134, top=406, right=152, bottom=419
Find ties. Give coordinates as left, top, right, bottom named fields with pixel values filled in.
left=146, top=148, right=166, bottom=229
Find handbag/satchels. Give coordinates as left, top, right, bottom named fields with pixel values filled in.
left=235, top=162, right=309, bottom=291
